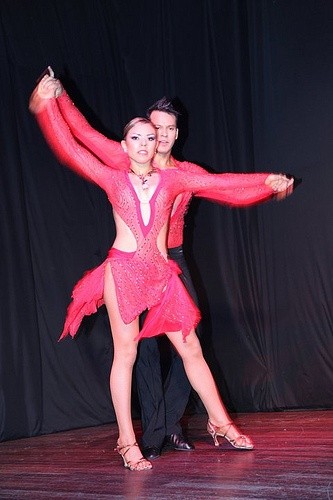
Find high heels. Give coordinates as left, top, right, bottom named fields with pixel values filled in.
left=116, top=438, right=153, bottom=471
left=206, top=420, right=254, bottom=450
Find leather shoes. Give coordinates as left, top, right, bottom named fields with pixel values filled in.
left=162, top=432, right=195, bottom=451
left=141, top=444, right=161, bottom=460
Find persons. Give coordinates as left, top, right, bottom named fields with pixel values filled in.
left=30, top=79, right=289, bottom=471
left=42, top=66, right=294, bottom=460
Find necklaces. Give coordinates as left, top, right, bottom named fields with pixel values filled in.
left=129, top=168, right=157, bottom=190
